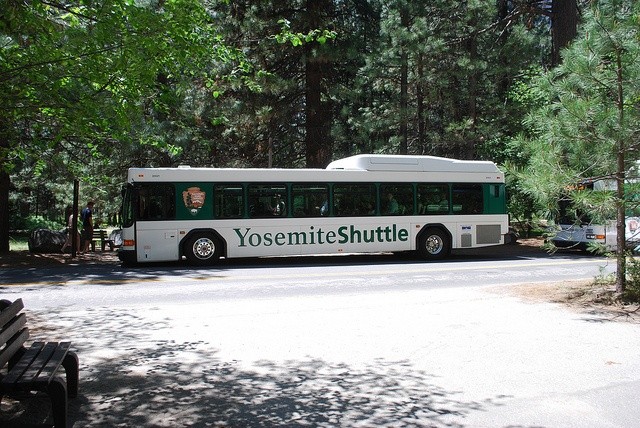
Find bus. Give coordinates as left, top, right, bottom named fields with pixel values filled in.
left=554, top=179, right=640, bottom=256
left=122, top=154, right=508, bottom=266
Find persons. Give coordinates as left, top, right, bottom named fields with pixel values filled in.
left=60, top=214, right=81, bottom=254
left=81, top=202, right=95, bottom=253
left=268, top=191, right=480, bottom=216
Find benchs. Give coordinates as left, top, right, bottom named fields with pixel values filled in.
left=88, top=229, right=115, bottom=251
left=0, top=298, right=80, bottom=427
